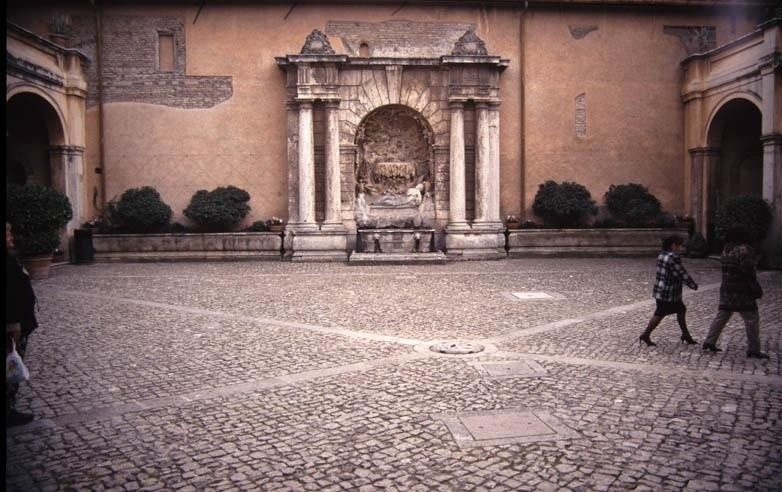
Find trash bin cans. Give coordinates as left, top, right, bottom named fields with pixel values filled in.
left=74, top=228, right=94, bottom=264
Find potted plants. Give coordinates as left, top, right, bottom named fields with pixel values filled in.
left=8, top=183, right=72, bottom=280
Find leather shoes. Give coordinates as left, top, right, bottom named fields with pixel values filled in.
left=746, top=349, right=770, bottom=359
left=8, top=412, right=34, bottom=427
left=701, top=342, right=723, bottom=352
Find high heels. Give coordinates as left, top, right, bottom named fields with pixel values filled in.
left=681, top=334, right=697, bottom=344
left=640, top=333, right=657, bottom=346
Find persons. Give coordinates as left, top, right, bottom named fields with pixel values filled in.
left=638, top=233, right=700, bottom=347
left=4, top=222, right=41, bottom=428
left=700, top=222, right=769, bottom=358
